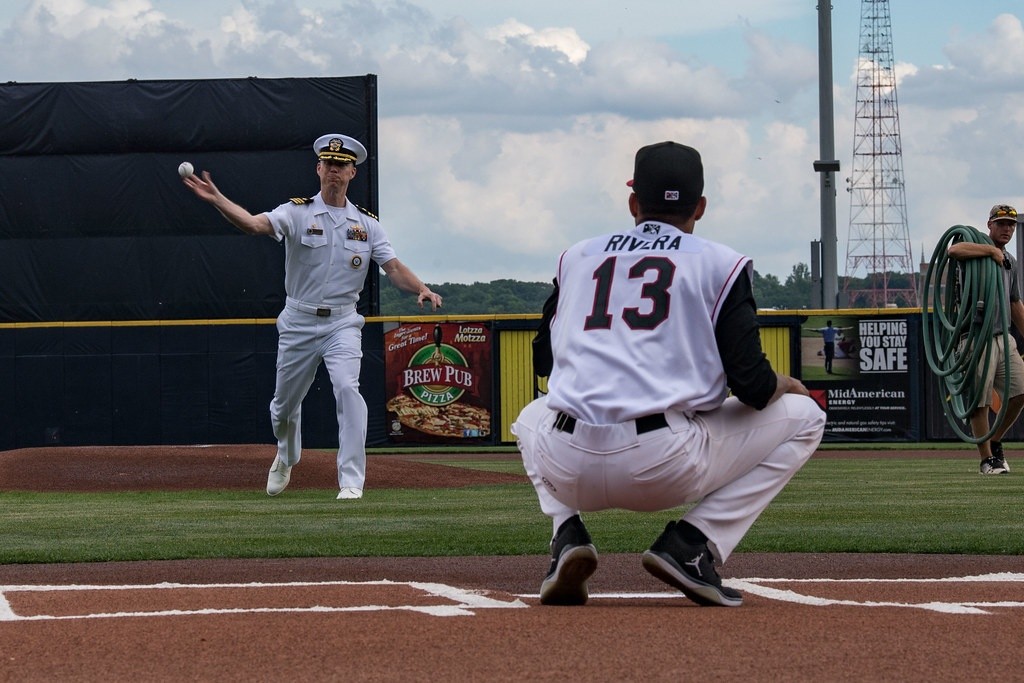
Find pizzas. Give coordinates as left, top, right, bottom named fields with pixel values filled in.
left=386, top=394, right=490, bottom=437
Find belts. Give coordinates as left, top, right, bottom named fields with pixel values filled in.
left=553, top=411, right=668, bottom=436
left=286, top=296, right=357, bottom=317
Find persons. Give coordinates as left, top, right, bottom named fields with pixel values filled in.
left=183, top=133, right=442, bottom=500
left=511, top=140, right=829, bottom=608
left=948, top=204, right=1023, bottom=476
left=834, top=327, right=844, bottom=341
left=802, top=320, right=855, bottom=375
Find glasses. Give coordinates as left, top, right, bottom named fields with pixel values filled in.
left=990, top=209, right=1017, bottom=221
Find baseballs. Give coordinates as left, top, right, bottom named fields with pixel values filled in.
left=177, top=161, right=195, bottom=179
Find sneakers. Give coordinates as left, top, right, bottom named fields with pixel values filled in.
left=978, top=440, right=1012, bottom=474
left=539, top=520, right=742, bottom=607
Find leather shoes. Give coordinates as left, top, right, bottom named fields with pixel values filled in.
left=336, top=485, right=363, bottom=501
left=266, top=453, right=292, bottom=495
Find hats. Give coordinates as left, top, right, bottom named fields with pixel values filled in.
left=313, top=134, right=368, bottom=165
left=627, top=141, right=704, bottom=190
left=989, top=204, right=1019, bottom=225
